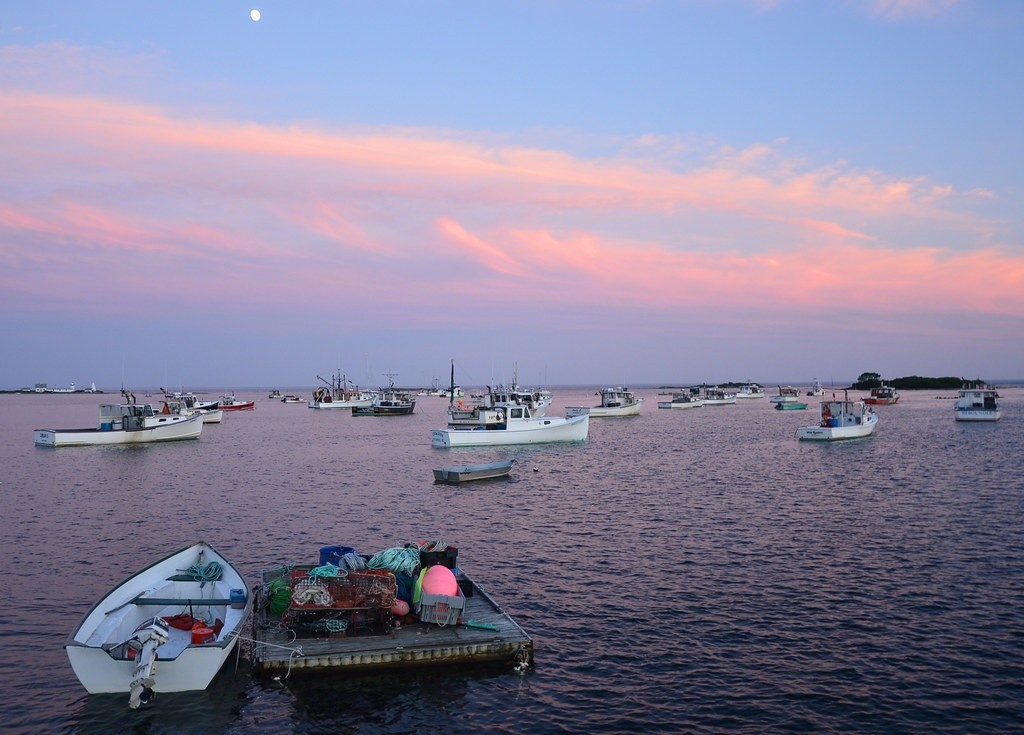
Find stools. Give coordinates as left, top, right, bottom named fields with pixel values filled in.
left=190, top=629, right=213, bottom=643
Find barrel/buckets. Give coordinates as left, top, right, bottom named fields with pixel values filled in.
left=319, top=546, right=355, bottom=567
left=191, top=628, right=214, bottom=644
left=101, top=422, right=112, bottom=431
left=827, top=417, right=838, bottom=427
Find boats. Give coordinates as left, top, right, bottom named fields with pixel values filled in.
left=62, top=540, right=254, bottom=708
left=34, top=386, right=205, bottom=448
left=218, top=391, right=255, bottom=411
left=432, top=459, right=516, bottom=483
left=954, top=383, right=1001, bottom=422
left=860, top=382, right=900, bottom=404
left=769, top=384, right=808, bottom=410
left=736, top=380, right=765, bottom=398
left=143, top=395, right=224, bottom=423
left=159, top=386, right=219, bottom=412
left=417, top=376, right=465, bottom=397
left=308, top=369, right=379, bottom=410
left=657, top=383, right=737, bottom=408
left=430, top=363, right=589, bottom=449
left=351, top=374, right=416, bottom=417
left=565, top=386, right=644, bottom=418
left=798, top=389, right=878, bottom=443
left=281, top=394, right=307, bottom=403
left=269, top=390, right=284, bottom=399
left=807, top=381, right=825, bottom=396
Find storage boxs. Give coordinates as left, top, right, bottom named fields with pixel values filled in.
left=230, top=588, right=245, bottom=608
left=286, top=544, right=473, bottom=635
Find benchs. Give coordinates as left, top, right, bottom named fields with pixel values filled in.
left=134, top=597, right=230, bottom=606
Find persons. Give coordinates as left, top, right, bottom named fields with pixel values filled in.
left=823, top=406, right=831, bottom=427
